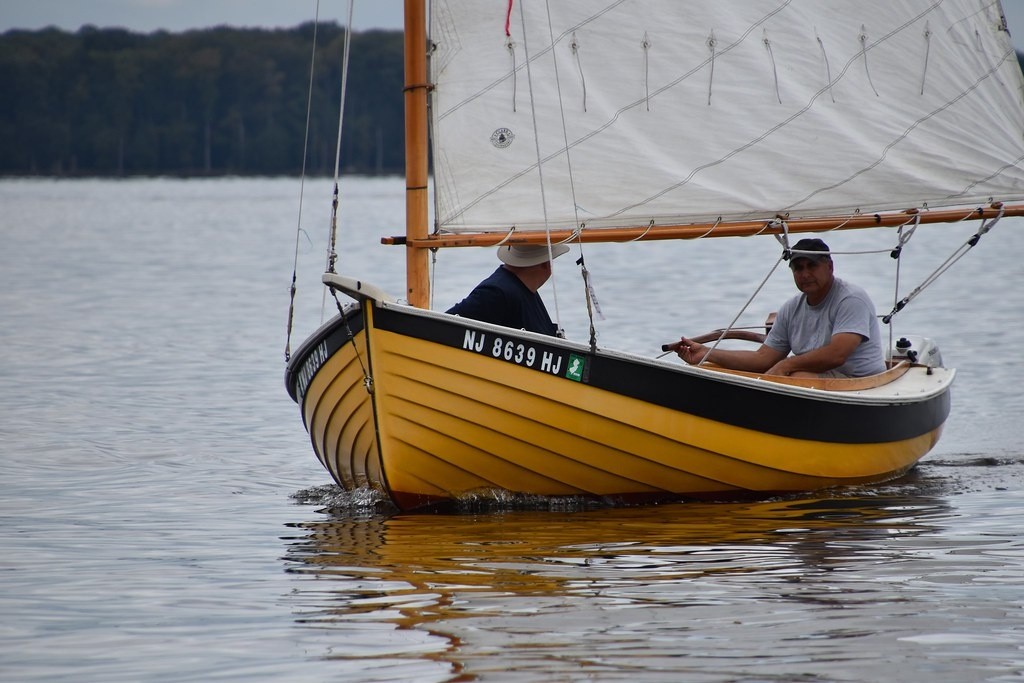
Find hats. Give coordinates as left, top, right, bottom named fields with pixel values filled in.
left=497, top=243, right=570, bottom=267
left=788, top=238, right=830, bottom=267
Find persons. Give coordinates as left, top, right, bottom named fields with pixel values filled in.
left=677, top=238, right=889, bottom=378
left=444, top=243, right=570, bottom=341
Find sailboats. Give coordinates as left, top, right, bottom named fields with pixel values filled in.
left=280, top=1, right=1024, bottom=515
